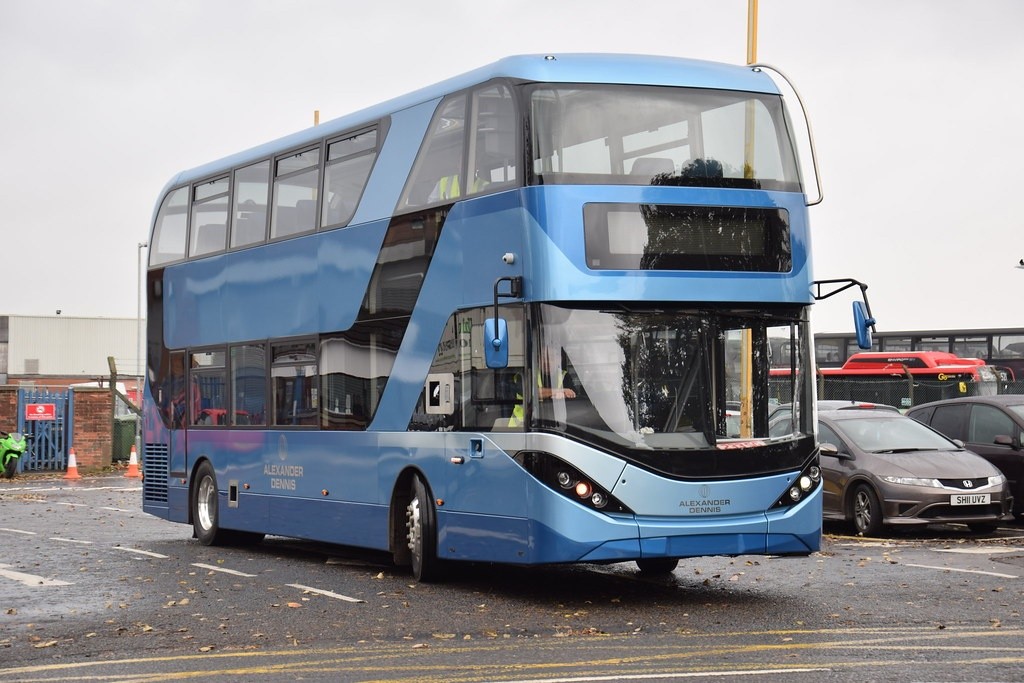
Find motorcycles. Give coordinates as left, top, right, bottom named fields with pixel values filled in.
left=0, top=430, right=33, bottom=479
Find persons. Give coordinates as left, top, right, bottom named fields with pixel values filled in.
left=426, top=155, right=489, bottom=206
left=508, top=341, right=577, bottom=428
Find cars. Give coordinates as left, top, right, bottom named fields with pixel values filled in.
left=726, top=398, right=1015, bottom=535
left=904, top=394, right=1024, bottom=520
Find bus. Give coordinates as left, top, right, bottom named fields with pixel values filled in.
left=736, top=327, right=1024, bottom=414
left=142, top=53, right=878, bottom=583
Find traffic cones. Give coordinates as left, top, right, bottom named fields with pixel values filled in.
left=124, top=444, right=141, bottom=477
left=62, top=447, right=82, bottom=478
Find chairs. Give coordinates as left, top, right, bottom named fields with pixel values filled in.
left=195, top=188, right=358, bottom=254
left=628, top=156, right=675, bottom=177
left=681, top=157, right=724, bottom=176
